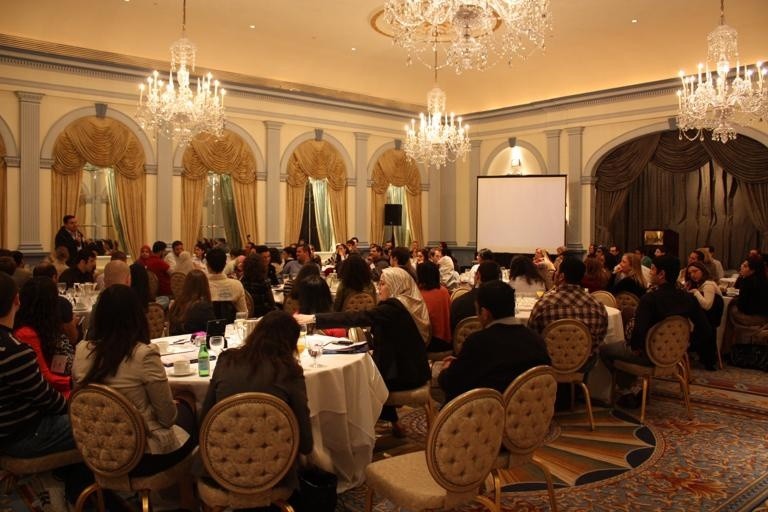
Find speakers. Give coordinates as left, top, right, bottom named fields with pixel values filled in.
left=384, top=203, right=402, bottom=226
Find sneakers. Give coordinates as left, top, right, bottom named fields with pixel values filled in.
left=615, top=384, right=641, bottom=395
left=391, top=425, right=406, bottom=438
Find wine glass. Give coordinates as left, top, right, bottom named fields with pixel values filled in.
left=209, top=335, right=224, bottom=364
left=295, top=334, right=307, bottom=366
left=299, top=323, right=308, bottom=342
left=536, top=288, right=546, bottom=300
left=57, top=282, right=67, bottom=296
left=308, top=339, right=325, bottom=370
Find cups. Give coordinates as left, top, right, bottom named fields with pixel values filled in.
left=236, top=311, right=249, bottom=326
left=283, top=274, right=290, bottom=285
left=173, top=359, right=190, bottom=374
left=156, top=341, right=168, bottom=353
left=332, top=279, right=339, bottom=289
left=465, top=268, right=471, bottom=279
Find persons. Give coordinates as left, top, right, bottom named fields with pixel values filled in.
left=0, top=239, right=767, bottom=512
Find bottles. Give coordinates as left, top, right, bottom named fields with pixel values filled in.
left=198, top=337, right=210, bottom=376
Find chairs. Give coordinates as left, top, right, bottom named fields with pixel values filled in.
left=490, top=365, right=558, bottom=512
left=365, top=386, right=507, bottom=512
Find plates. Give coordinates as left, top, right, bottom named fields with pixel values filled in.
left=168, top=368, right=198, bottom=377
left=160, top=347, right=175, bottom=356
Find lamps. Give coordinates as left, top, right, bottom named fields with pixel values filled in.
left=384, top=0, right=553, bottom=76
left=675, top=0, right=768, bottom=143
left=402, top=34, right=471, bottom=171
left=133, top=0, right=226, bottom=148
